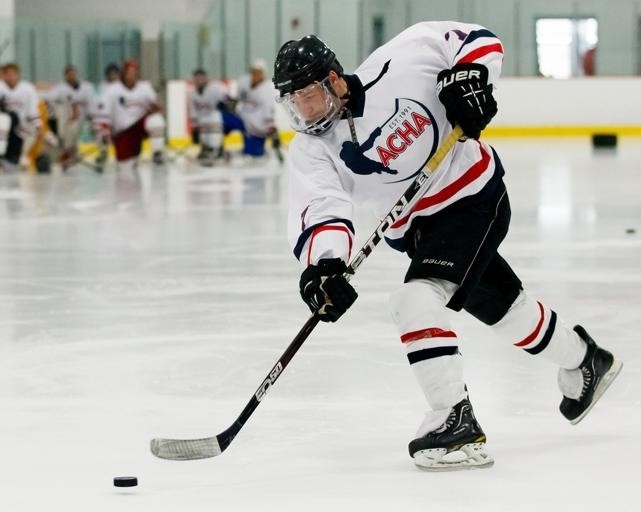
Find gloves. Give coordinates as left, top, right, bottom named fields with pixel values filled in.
left=435, top=63, right=496, bottom=139
left=299, top=258, right=357, bottom=322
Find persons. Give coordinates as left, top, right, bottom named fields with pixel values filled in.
left=111, top=62, right=172, bottom=172
left=272, top=20, right=613, bottom=458
left=187, top=70, right=229, bottom=166
left=0, top=61, right=58, bottom=170
left=101, top=61, right=121, bottom=94
left=50, top=64, right=97, bottom=148
left=217, top=61, right=277, bottom=155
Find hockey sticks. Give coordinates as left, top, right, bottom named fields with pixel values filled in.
left=150, top=124, right=465, bottom=461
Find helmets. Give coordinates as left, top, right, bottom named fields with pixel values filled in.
left=273, top=35, right=345, bottom=136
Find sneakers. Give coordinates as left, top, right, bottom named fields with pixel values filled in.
left=410, top=387, right=486, bottom=456
left=558, top=326, right=614, bottom=419
